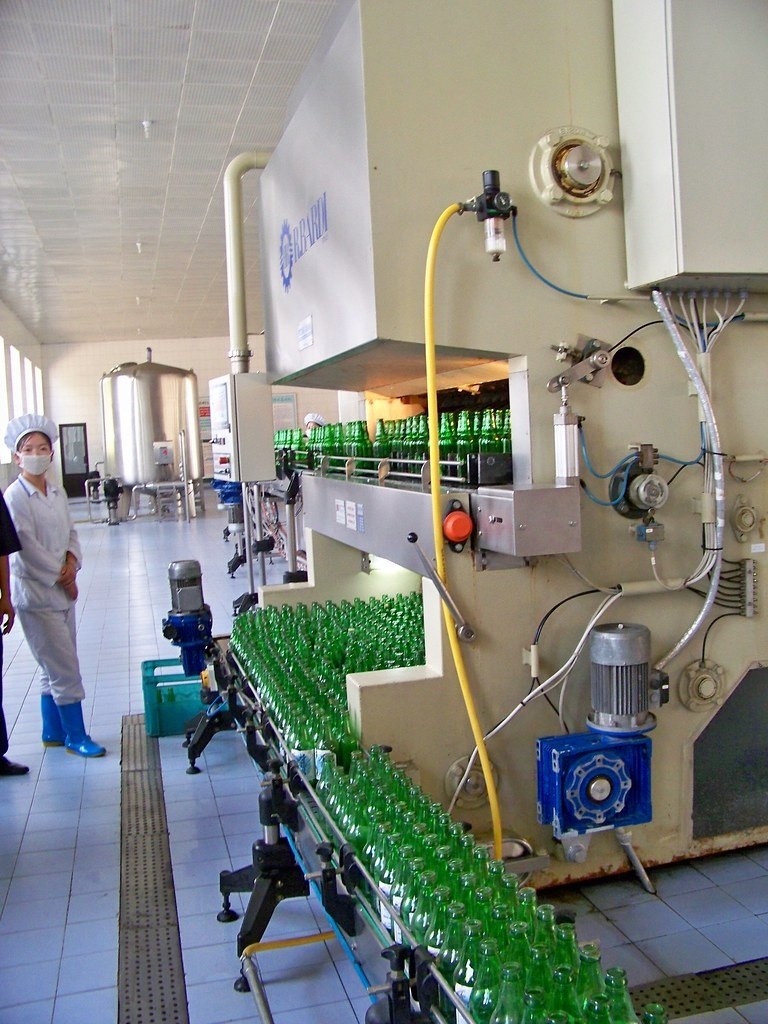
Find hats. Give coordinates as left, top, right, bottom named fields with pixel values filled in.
left=304, top=413, right=328, bottom=427
left=3, top=413, right=58, bottom=452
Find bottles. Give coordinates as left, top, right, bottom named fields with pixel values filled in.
left=273, top=409, right=513, bottom=486
left=230, top=591, right=668, bottom=1024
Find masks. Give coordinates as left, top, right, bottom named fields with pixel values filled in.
left=15, top=452, right=53, bottom=475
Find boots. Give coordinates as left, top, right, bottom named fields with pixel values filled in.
left=40, top=694, right=67, bottom=747
left=55, top=701, right=106, bottom=757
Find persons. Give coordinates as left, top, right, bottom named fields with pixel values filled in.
left=3, top=414, right=107, bottom=758
left=304, top=414, right=326, bottom=441
left=0, top=490, right=30, bottom=775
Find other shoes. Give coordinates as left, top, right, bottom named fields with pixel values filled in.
left=0, top=756, right=29, bottom=776
